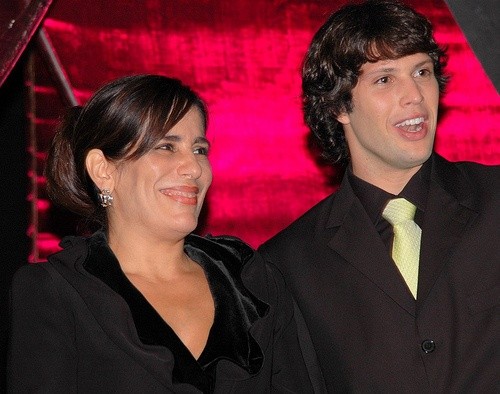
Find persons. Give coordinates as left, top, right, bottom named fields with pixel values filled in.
left=0, top=75, right=327, bottom=394
left=257, top=1, right=500, bottom=394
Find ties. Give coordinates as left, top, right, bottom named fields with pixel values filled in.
left=381, top=198, right=422, bottom=300
